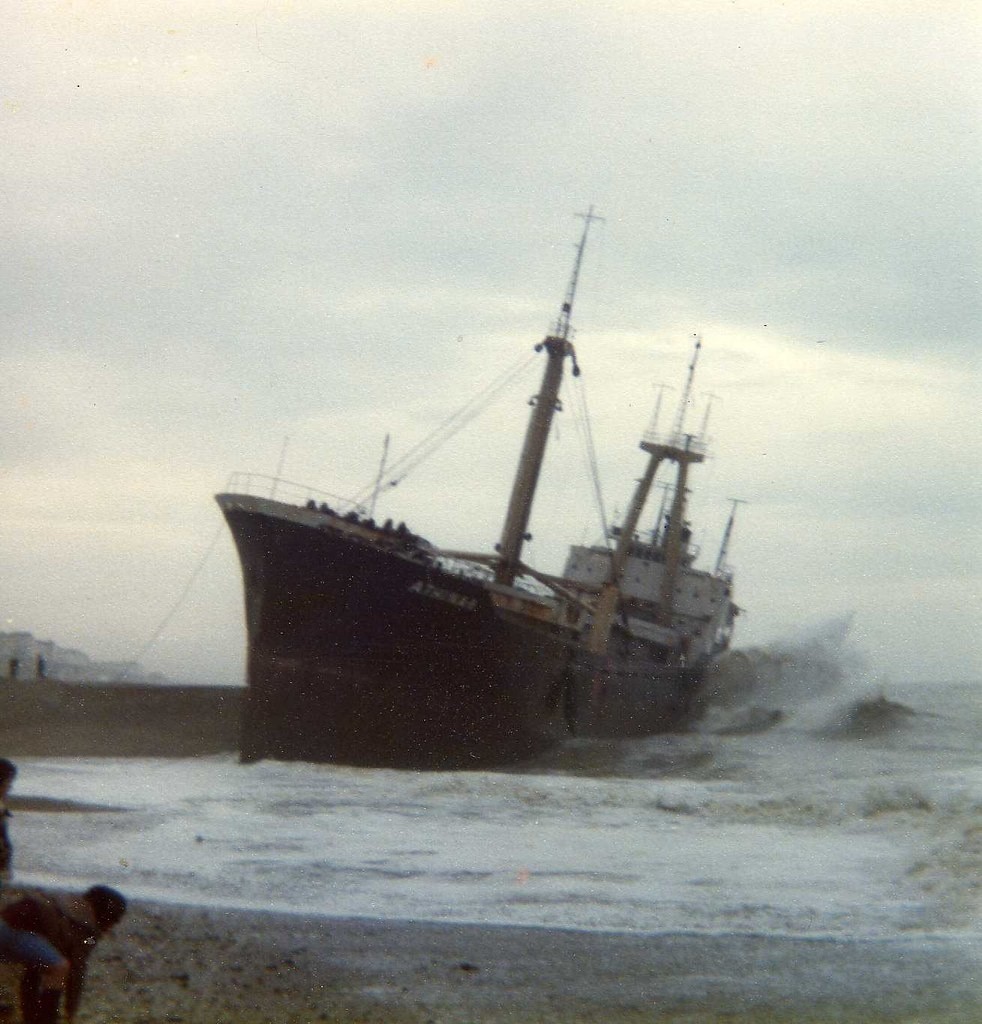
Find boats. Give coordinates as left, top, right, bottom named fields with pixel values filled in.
left=212, top=204, right=749, bottom=763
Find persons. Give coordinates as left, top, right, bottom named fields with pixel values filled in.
left=0, top=884, right=128, bottom=1024
left=0, top=756, right=17, bottom=884
